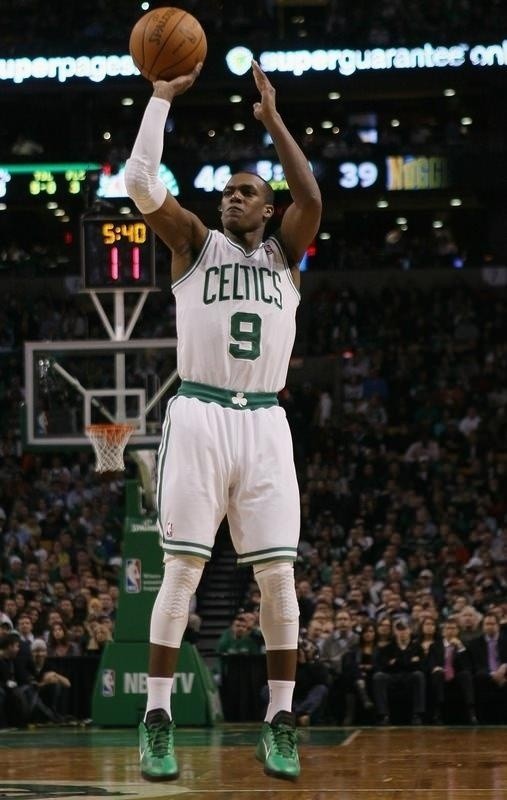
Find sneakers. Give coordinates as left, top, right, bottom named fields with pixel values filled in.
left=138, top=708, right=180, bottom=782
left=253, top=710, right=303, bottom=781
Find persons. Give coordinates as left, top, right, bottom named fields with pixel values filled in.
left=123, top=59, right=323, bottom=782
left=1, top=239, right=182, bottom=730
left=1, top=1, right=507, bottom=270
left=220, top=278, right=506, bottom=725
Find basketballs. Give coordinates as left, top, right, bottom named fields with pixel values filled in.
left=131, top=8, right=207, bottom=82
